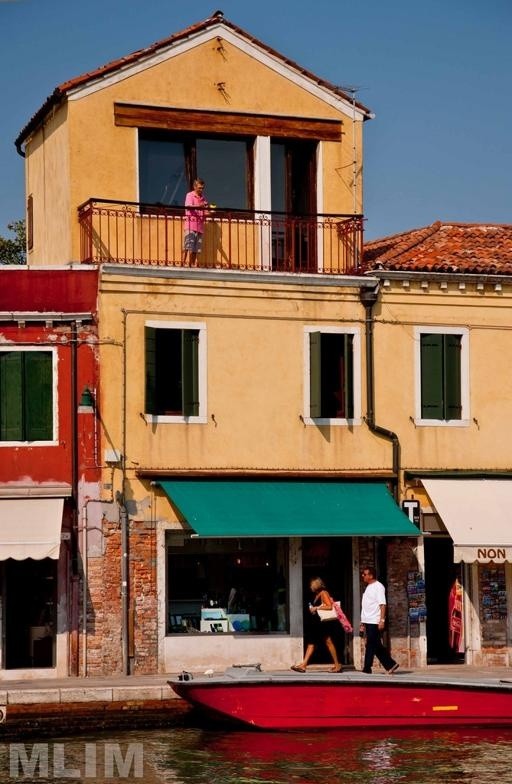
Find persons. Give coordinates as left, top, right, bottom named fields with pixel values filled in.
left=290, top=578, right=343, bottom=673
left=239, top=573, right=279, bottom=632
left=360, top=567, right=400, bottom=675
left=181, top=177, right=210, bottom=266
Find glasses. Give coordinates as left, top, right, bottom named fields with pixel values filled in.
left=363, top=573, right=369, bottom=576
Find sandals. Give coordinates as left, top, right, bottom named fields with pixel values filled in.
left=328, top=663, right=342, bottom=673
left=290, top=663, right=307, bottom=673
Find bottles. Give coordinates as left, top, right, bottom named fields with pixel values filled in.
left=308, top=602, right=316, bottom=615
left=359, top=623, right=365, bottom=638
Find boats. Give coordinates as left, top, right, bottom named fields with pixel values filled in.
left=166, top=665, right=512, bottom=731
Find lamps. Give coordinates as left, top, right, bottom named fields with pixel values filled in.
left=78, top=386, right=98, bottom=416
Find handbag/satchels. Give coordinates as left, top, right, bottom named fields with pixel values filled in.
left=315, top=592, right=341, bottom=622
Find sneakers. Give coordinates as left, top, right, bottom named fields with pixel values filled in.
left=389, top=663, right=399, bottom=673
